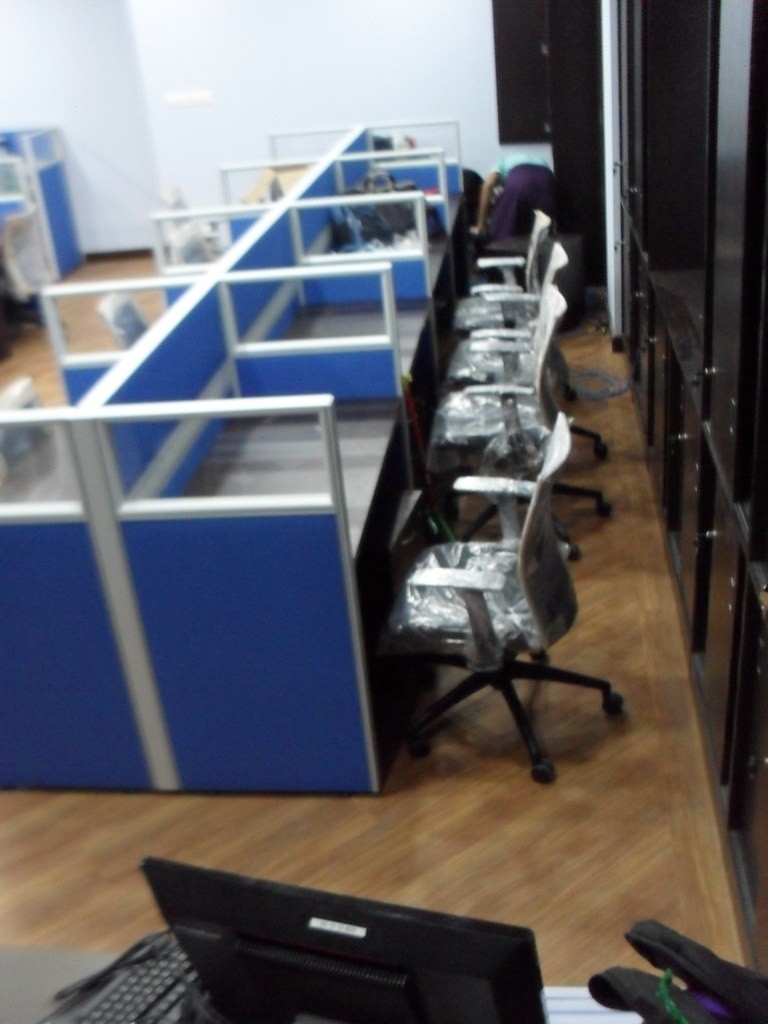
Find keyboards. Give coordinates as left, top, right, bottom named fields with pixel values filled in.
left=38, top=928, right=211, bottom=1024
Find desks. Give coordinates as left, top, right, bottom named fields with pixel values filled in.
left=0, top=946, right=640, bottom=1024
left=2, top=125, right=477, bottom=796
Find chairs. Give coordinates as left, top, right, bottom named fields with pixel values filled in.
left=392, top=213, right=619, bottom=784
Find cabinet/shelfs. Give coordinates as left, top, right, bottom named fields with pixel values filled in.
left=492, top=0, right=602, bottom=285
left=616, top=1, right=765, bottom=991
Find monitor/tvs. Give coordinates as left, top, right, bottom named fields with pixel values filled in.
left=138, top=856, right=549, bottom=1024
